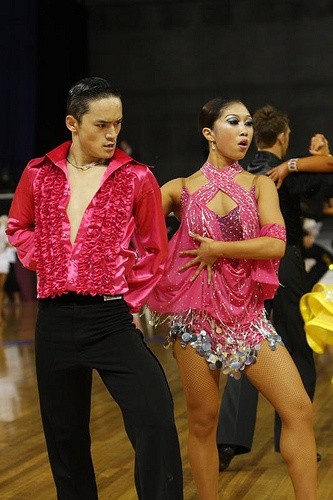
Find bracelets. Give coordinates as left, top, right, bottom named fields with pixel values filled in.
left=288, top=158, right=298, bottom=171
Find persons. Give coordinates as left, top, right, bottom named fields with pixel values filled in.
left=161, top=97, right=317, bottom=500
left=5, top=78, right=185, bottom=500
left=266, top=133, right=333, bottom=354
left=215, top=106, right=333, bottom=472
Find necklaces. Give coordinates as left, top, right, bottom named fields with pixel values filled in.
left=67, top=157, right=105, bottom=170
